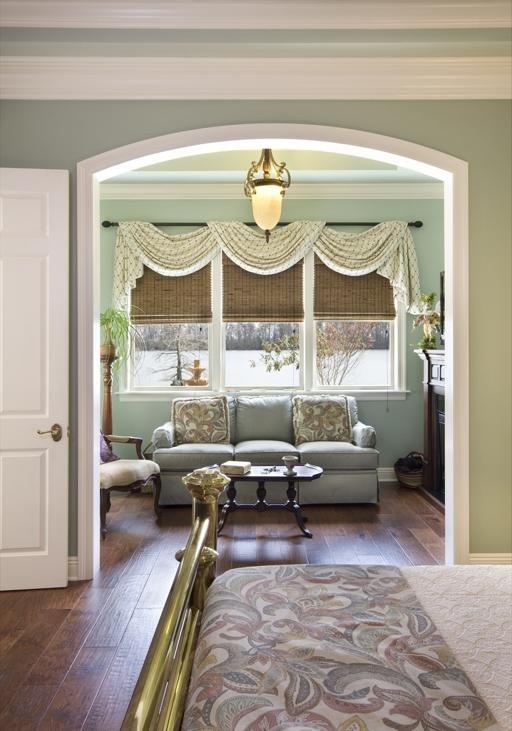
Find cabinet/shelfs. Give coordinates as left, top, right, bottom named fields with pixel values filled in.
left=417, top=349, right=446, bottom=513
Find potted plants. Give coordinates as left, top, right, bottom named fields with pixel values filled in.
left=100, top=301, right=146, bottom=372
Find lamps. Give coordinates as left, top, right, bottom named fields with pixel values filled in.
left=245, top=149, right=293, bottom=244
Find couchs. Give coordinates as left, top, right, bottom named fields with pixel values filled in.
left=148, top=393, right=380, bottom=511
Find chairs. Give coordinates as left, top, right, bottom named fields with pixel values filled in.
left=99, top=429, right=162, bottom=533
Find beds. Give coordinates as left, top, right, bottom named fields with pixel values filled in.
left=112, top=467, right=511, bottom=731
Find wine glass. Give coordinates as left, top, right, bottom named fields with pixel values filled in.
left=282, top=455, right=298, bottom=476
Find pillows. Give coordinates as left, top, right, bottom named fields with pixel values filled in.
left=172, top=396, right=230, bottom=444
left=293, top=393, right=352, bottom=444
left=99, top=430, right=119, bottom=464
